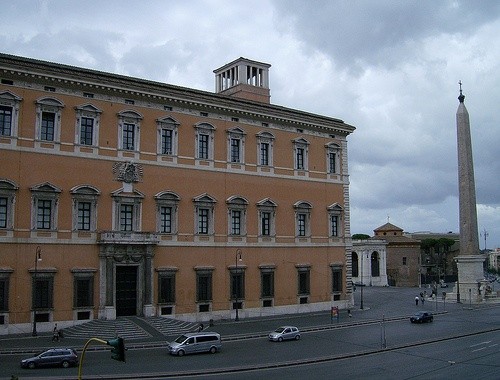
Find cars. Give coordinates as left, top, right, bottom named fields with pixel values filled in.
left=410, top=311, right=433, bottom=324
left=269, top=326, right=301, bottom=342
left=21, top=349, right=78, bottom=368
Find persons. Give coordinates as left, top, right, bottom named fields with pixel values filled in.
left=51, top=327, right=60, bottom=342
left=353, top=284, right=356, bottom=291
left=483, top=271, right=498, bottom=284
left=415, top=279, right=444, bottom=305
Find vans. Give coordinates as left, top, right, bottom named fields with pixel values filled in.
left=168, top=332, right=222, bottom=356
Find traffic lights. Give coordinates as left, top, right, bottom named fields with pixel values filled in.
left=107, top=337, right=126, bottom=363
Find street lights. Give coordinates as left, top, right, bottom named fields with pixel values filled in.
left=480, top=228, right=490, bottom=277
left=360, top=249, right=371, bottom=309
left=32, top=246, right=42, bottom=336
left=235, top=250, right=243, bottom=321
